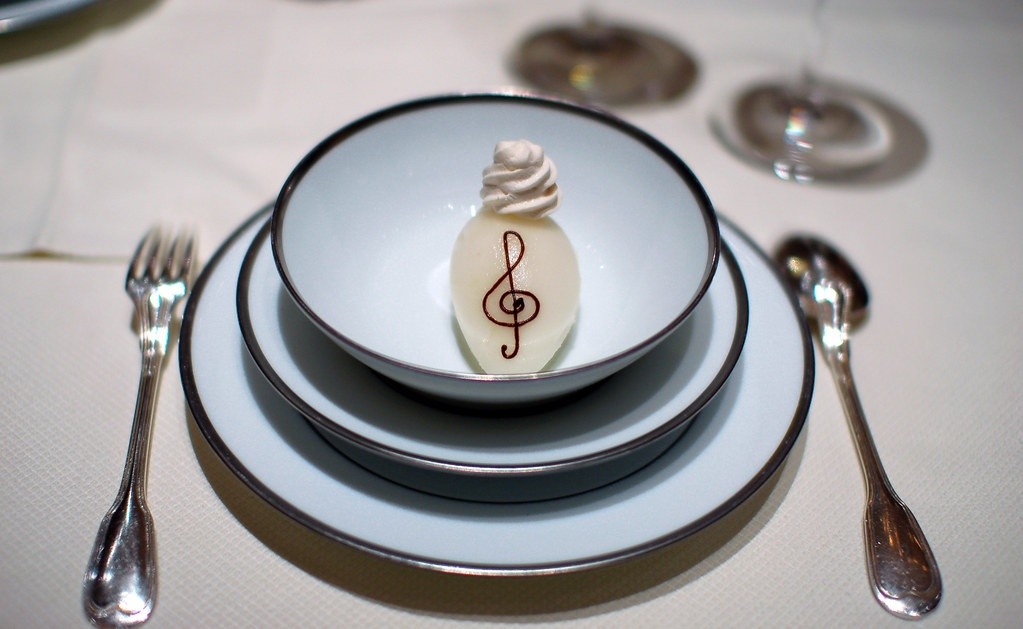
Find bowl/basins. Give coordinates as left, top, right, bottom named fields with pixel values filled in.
left=269, top=91, right=722, bottom=401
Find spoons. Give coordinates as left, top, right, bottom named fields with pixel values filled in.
left=772, top=232, right=943, bottom=622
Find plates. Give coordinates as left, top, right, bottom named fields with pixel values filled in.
left=0, top=0, right=91, bottom=32
left=177, top=198, right=818, bottom=576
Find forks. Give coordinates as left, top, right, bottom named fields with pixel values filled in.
left=82, top=225, right=199, bottom=626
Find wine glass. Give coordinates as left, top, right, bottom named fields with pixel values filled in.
left=704, top=0, right=928, bottom=184
left=503, top=0, right=700, bottom=106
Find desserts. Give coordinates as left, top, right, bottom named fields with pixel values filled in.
left=449, top=138, right=579, bottom=375
